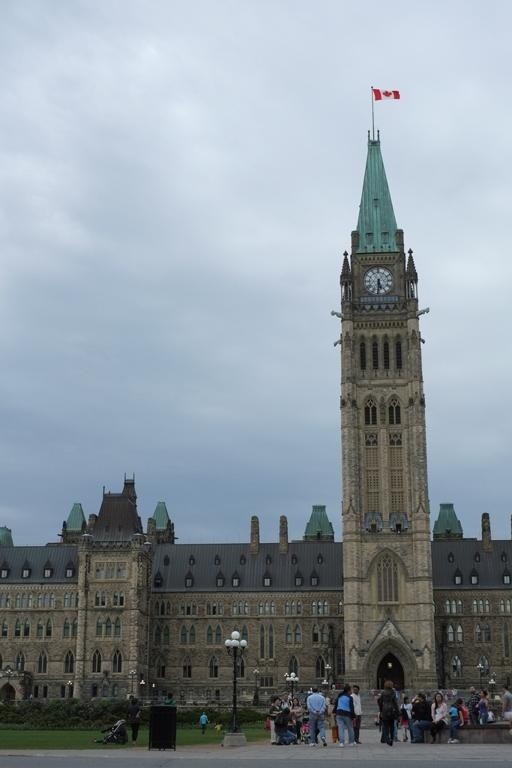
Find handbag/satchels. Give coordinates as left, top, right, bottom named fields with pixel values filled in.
left=264, top=720, right=271, bottom=730
left=331, top=726, right=339, bottom=739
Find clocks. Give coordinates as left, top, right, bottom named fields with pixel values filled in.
left=363, top=264, right=396, bottom=297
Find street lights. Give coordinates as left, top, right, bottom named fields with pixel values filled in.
left=489, top=679, right=496, bottom=694
left=139, top=679, right=146, bottom=705
left=252, top=668, right=260, bottom=700
left=221, top=630, right=249, bottom=733
left=280, top=661, right=332, bottom=705
left=476, top=663, right=485, bottom=691
left=129, top=670, right=137, bottom=696
left=66, top=680, right=74, bottom=699
left=6, top=669, right=13, bottom=702
left=151, top=683, right=156, bottom=702
left=491, top=671, right=497, bottom=688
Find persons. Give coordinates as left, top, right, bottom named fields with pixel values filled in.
left=215, top=723, right=223, bottom=737
left=199, top=712, right=208, bottom=735
left=127, top=698, right=141, bottom=745
left=268, top=681, right=496, bottom=746
left=501, top=684, right=512, bottom=721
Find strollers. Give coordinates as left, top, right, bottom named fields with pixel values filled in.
left=300, top=710, right=311, bottom=745
left=97, top=717, right=128, bottom=746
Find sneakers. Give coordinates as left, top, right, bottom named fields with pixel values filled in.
left=269, top=736, right=460, bottom=749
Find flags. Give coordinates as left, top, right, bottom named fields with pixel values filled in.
left=372, top=88, right=400, bottom=102
